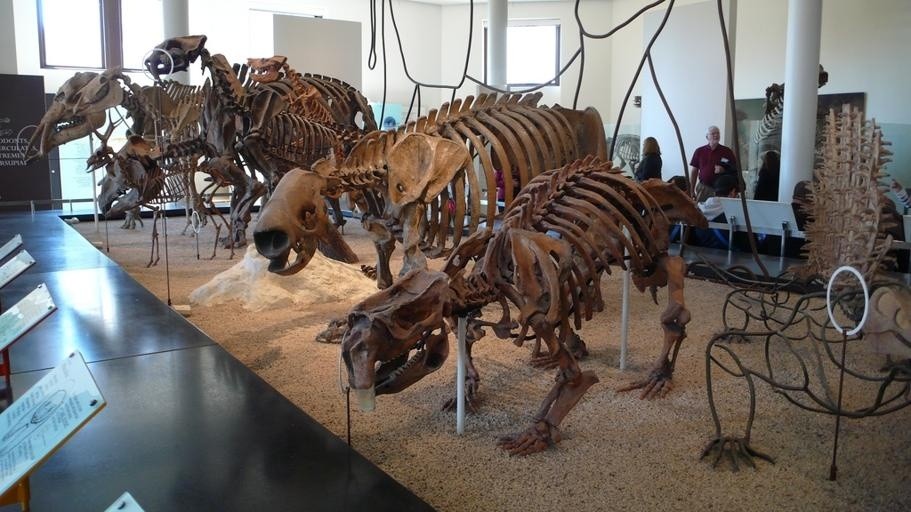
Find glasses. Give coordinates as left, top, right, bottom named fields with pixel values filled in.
left=737, top=194, right=739, bottom=198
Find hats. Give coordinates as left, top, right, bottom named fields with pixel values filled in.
left=714, top=176, right=737, bottom=193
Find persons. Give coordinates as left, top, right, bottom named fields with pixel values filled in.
left=754, top=151, right=780, bottom=201
left=667, top=176, right=689, bottom=196
left=495, top=167, right=520, bottom=213
left=634, top=137, right=662, bottom=182
left=890, top=179, right=911, bottom=208
left=690, top=126, right=737, bottom=201
left=687, top=175, right=762, bottom=253
